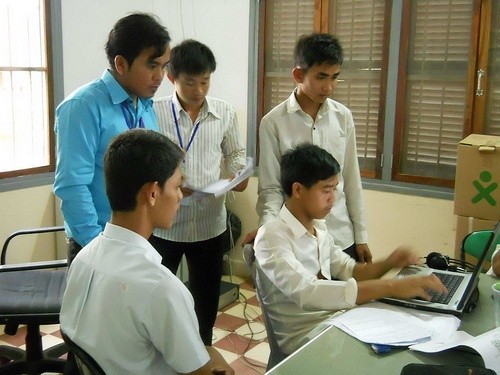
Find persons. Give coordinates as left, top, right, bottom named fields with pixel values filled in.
left=241, top=32, right=372, bottom=264
left=52, top=14, right=170, bottom=375
left=149, top=41, right=249, bottom=347
left=253, top=142, right=449, bottom=355
left=59, top=131, right=236, bottom=375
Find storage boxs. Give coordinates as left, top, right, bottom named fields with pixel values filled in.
left=452, top=133, right=499, bottom=272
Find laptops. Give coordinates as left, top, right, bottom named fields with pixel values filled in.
left=377, top=219, right=500, bottom=315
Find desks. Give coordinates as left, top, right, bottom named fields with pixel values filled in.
left=259, top=250, right=500, bottom=375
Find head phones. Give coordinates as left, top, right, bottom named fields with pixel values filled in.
left=426, top=252, right=476, bottom=272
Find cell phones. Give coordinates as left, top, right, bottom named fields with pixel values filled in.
left=371, top=344, right=391, bottom=353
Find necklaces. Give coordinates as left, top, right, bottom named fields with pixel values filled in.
left=122, top=103, right=145, bottom=131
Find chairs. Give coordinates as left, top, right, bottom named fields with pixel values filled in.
left=0, top=217, right=84, bottom=375
